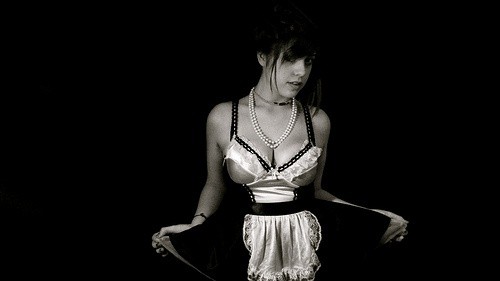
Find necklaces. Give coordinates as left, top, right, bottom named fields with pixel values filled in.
left=249, top=86, right=298, bottom=148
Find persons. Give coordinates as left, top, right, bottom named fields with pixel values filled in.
left=151, top=1, right=410, bottom=281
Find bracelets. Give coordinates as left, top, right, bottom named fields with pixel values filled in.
left=193, top=213, right=207, bottom=220
